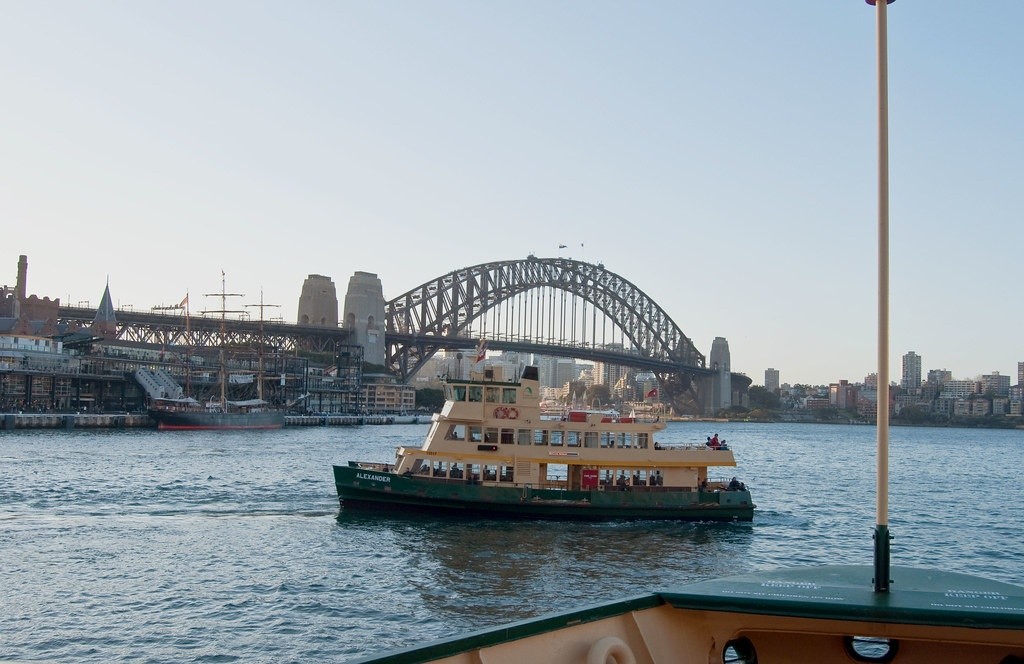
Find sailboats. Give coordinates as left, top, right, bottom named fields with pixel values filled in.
left=145, top=269, right=312, bottom=434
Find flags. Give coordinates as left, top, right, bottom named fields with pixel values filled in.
left=476, top=341, right=489, bottom=362
left=644, top=387, right=659, bottom=398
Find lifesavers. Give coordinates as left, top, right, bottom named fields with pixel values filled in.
left=495, top=407, right=507, bottom=419
left=506, top=407, right=519, bottom=419
left=62, top=420, right=65, bottom=425
left=114, top=419, right=118, bottom=425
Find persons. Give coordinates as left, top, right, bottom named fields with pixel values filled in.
left=403, top=467, right=414, bottom=479
left=654, top=442, right=662, bottom=450
left=453, top=432, right=457, bottom=438
left=618, top=475, right=642, bottom=485
left=705, top=433, right=729, bottom=450
left=610, top=440, right=615, bottom=448
left=699, top=480, right=710, bottom=492
left=648, top=469, right=663, bottom=486
left=449, top=464, right=463, bottom=479
left=728, top=477, right=746, bottom=491
left=483, top=464, right=506, bottom=481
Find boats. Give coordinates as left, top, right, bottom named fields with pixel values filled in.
left=332, top=336, right=758, bottom=524
